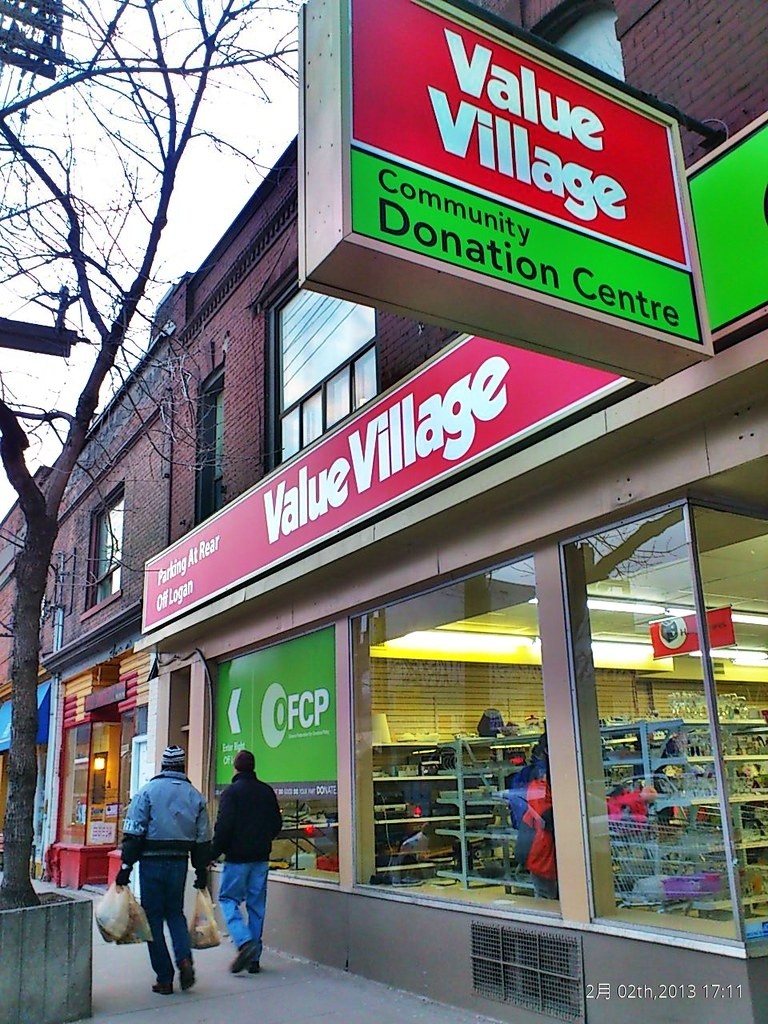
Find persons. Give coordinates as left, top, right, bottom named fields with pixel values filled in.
left=115, top=743, right=213, bottom=997
left=211, top=749, right=284, bottom=975
left=508, top=733, right=559, bottom=900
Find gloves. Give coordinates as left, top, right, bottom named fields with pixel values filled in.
left=116, top=866, right=132, bottom=888
left=193, top=869, right=209, bottom=889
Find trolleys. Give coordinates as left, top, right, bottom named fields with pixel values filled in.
left=608, top=820, right=730, bottom=914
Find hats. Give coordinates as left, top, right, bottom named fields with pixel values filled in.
left=234, top=749, right=254, bottom=772
left=160, top=744, right=185, bottom=767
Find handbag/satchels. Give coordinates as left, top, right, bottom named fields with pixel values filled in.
left=94, top=882, right=129, bottom=942
left=114, top=884, right=153, bottom=945
left=188, top=887, right=222, bottom=949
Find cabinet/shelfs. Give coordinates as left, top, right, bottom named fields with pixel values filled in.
left=434, top=734, right=542, bottom=899
left=647, top=716, right=768, bottom=919
left=599, top=719, right=662, bottom=915
left=371, top=740, right=493, bottom=872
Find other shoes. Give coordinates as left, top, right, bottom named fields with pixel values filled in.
left=151, top=982, right=174, bottom=995
left=246, top=961, right=260, bottom=974
left=230, top=940, right=259, bottom=974
left=178, top=965, right=195, bottom=990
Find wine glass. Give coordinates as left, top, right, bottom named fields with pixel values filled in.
left=689, top=731, right=736, bottom=757
left=667, top=691, right=747, bottom=720
left=674, top=772, right=757, bottom=796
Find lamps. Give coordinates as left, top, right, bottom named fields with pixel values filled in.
left=530, top=596, right=768, bottom=625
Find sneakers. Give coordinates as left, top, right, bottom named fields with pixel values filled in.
left=392, top=875, right=423, bottom=887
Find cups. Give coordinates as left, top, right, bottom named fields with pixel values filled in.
left=749, top=704, right=760, bottom=719
